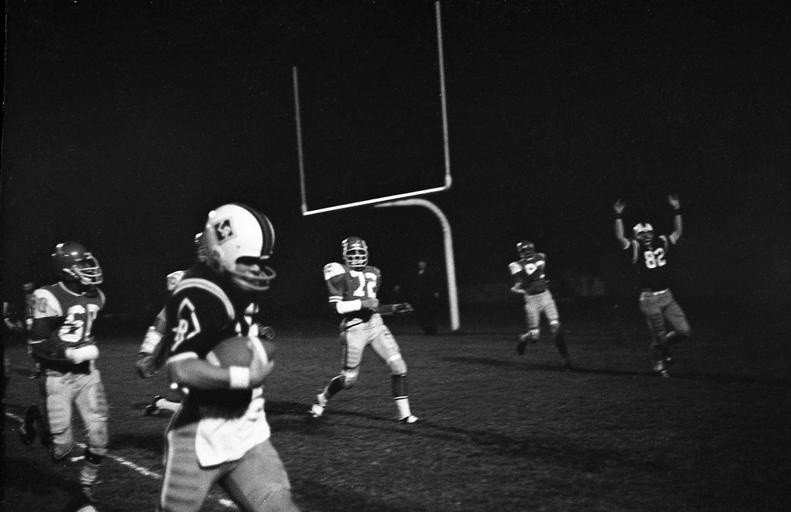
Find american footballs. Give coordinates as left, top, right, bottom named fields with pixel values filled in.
left=205, top=335, right=275, bottom=368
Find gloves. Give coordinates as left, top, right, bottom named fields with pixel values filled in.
left=66, top=344, right=98, bottom=364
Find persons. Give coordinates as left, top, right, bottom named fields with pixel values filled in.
left=135, top=233, right=274, bottom=415
left=158, top=202, right=301, bottom=512
left=410, top=258, right=439, bottom=332
left=508, top=240, right=561, bottom=356
left=306, top=236, right=425, bottom=425
left=20, top=242, right=110, bottom=505
left=610, top=192, right=692, bottom=377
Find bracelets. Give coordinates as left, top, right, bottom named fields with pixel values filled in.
left=672, top=208, right=683, bottom=216
left=228, top=364, right=250, bottom=392
left=611, top=213, right=625, bottom=219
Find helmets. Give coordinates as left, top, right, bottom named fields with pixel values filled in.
left=52, top=243, right=104, bottom=286
left=195, top=204, right=275, bottom=290
left=517, top=242, right=534, bottom=258
left=633, top=223, right=654, bottom=245
left=342, top=236, right=368, bottom=271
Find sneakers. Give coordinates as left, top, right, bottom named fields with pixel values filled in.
left=655, top=365, right=669, bottom=377
left=147, top=396, right=163, bottom=415
left=308, top=394, right=326, bottom=418
left=398, top=414, right=420, bottom=424
left=21, top=405, right=38, bottom=444
